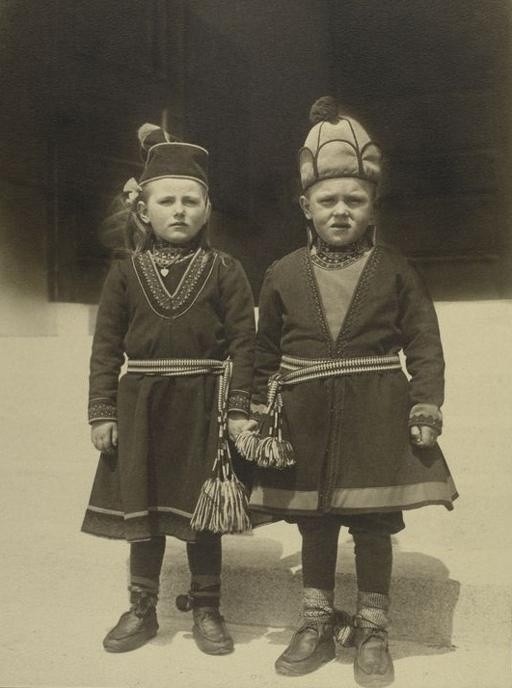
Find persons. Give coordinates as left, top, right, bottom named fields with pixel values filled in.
left=257, top=95, right=460, bottom=688
left=77, top=117, right=256, bottom=656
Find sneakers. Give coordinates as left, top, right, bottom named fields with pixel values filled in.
left=192, top=605, right=234, bottom=654
left=352, top=629, right=393, bottom=688
left=274, top=619, right=336, bottom=675
left=104, top=610, right=158, bottom=654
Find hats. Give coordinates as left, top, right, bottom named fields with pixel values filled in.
left=299, top=96, right=383, bottom=189
left=138, top=123, right=209, bottom=190
